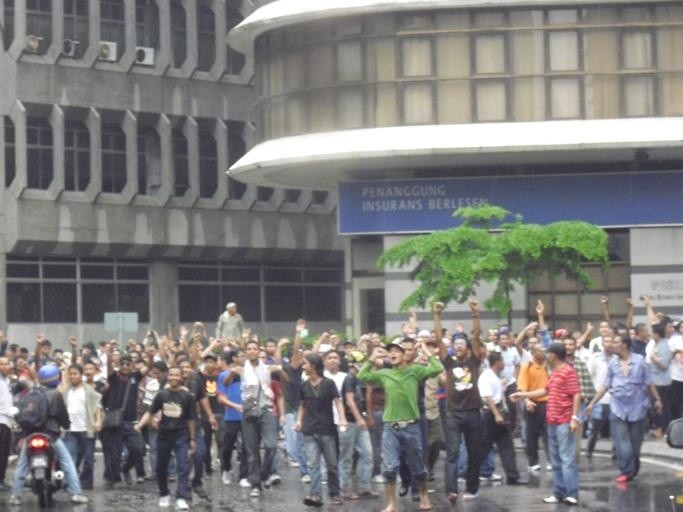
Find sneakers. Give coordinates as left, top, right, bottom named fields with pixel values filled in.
left=462, top=490, right=480, bottom=501
left=193, top=485, right=213, bottom=502
left=158, top=494, right=170, bottom=508
left=0, top=479, right=13, bottom=492
left=8, top=494, right=23, bottom=505
left=276, top=444, right=312, bottom=483
left=615, top=473, right=631, bottom=483
left=453, top=462, right=555, bottom=482
left=543, top=496, right=561, bottom=504
left=221, top=470, right=281, bottom=497
left=104, top=468, right=176, bottom=491
left=562, top=496, right=577, bottom=505
left=174, top=497, right=189, bottom=511
left=447, top=492, right=458, bottom=504
left=70, top=493, right=89, bottom=504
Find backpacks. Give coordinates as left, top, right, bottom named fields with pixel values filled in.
left=16, top=387, right=49, bottom=431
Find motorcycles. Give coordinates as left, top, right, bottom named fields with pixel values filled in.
left=16, top=428, right=68, bottom=512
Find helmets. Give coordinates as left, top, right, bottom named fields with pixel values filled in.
left=39, top=364, right=62, bottom=387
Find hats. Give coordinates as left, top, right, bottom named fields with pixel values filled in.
left=386, top=342, right=406, bottom=354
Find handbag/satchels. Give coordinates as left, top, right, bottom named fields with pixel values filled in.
left=101, top=407, right=125, bottom=428
left=242, top=396, right=264, bottom=420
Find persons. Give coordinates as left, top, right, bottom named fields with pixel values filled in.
left=0, top=294, right=683, bottom=511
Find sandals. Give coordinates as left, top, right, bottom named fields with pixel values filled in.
left=328, top=487, right=380, bottom=505
left=304, top=496, right=323, bottom=508
left=418, top=492, right=432, bottom=510
left=378, top=504, right=397, bottom=512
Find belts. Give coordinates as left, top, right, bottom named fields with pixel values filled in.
left=388, top=419, right=418, bottom=431
left=260, top=404, right=274, bottom=412
left=122, top=417, right=138, bottom=423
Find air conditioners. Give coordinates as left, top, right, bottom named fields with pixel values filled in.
left=98, top=41, right=118, bottom=62
left=25, top=34, right=46, bottom=55
left=61, top=38, right=80, bottom=59
left=135, top=45, right=155, bottom=67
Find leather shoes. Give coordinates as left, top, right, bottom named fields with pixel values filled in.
left=398, top=479, right=420, bottom=502
